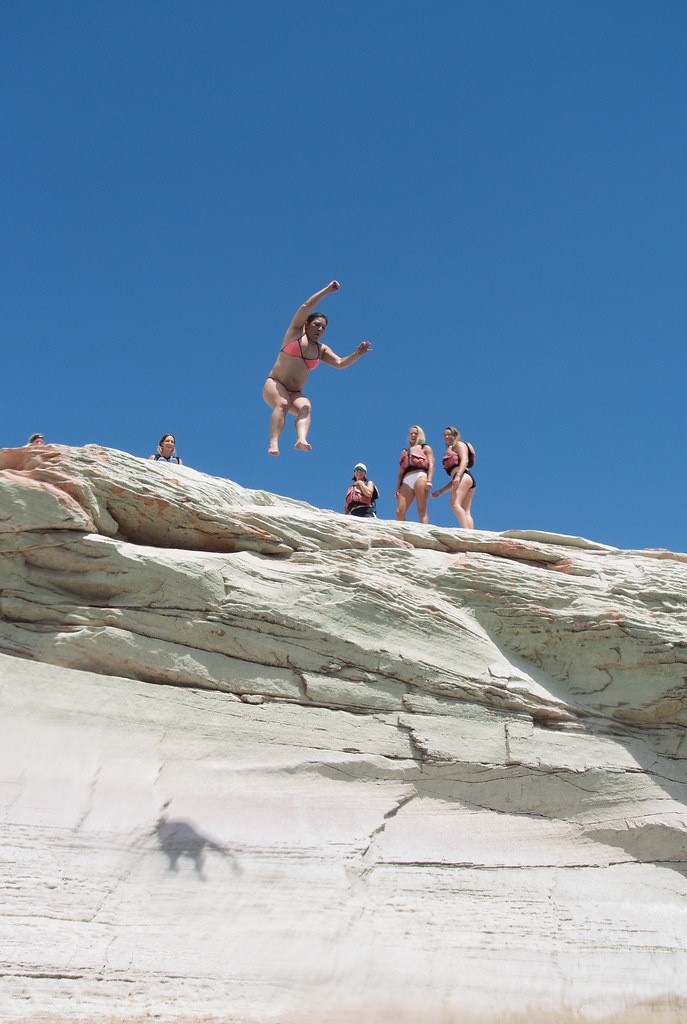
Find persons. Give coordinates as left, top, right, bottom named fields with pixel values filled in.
left=22, top=433, right=45, bottom=447
left=432, top=425, right=477, bottom=529
left=395, top=424, right=434, bottom=524
left=148, top=433, right=182, bottom=464
left=262, top=280, right=372, bottom=456
left=344, top=463, right=379, bottom=517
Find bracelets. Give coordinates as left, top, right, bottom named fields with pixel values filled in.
left=456, top=473, right=462, bottom=477
left=440, top=490, right=443, bottom=493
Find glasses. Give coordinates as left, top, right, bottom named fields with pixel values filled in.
left=355, top=467, right=365, bottom=472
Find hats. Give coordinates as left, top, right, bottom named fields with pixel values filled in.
left=354, top=463, right=367, bottom=473
